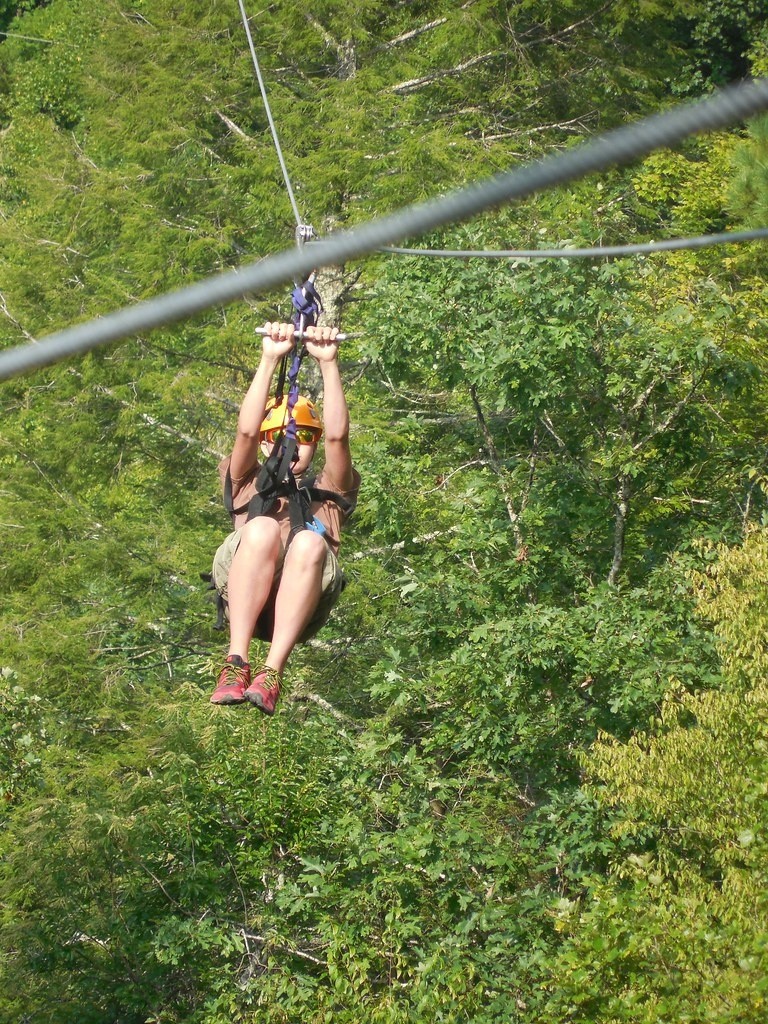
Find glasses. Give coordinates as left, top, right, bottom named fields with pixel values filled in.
left=260, top=427, right=319, bottom=447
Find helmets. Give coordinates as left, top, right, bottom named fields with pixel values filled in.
left=261, top=395, right=323, bottom=431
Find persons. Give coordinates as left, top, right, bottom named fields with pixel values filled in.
left=211, top=321, right=361, bottom=716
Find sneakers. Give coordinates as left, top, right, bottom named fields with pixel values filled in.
left=243, top=664, right=289, bottom=718
left=210, top=655, right=251, bottom=705
